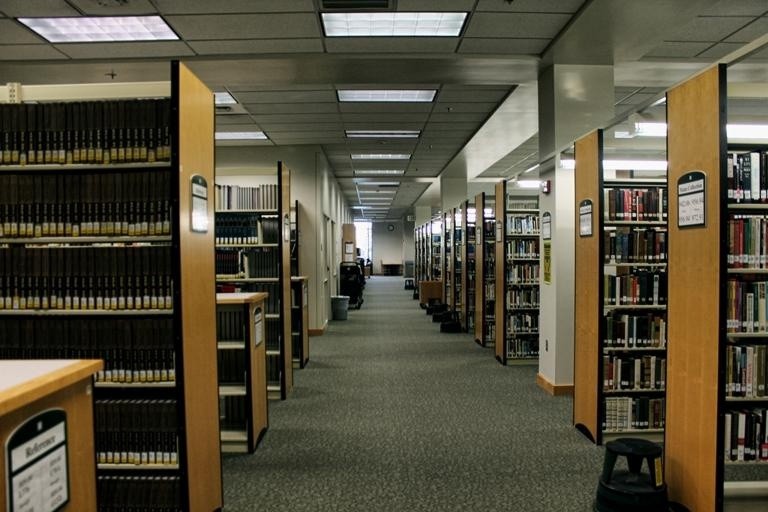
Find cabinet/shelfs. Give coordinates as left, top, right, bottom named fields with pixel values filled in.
left=574, top=130, right=669, bottom=445
left=0, top=358, right=106, bottom=512
left=0, top=58, right=225, bottom=512
left=665, top=64, right=767, bottom=510
left=416, top=181, right=542, bottom=366
left=212, top=159, right=307, bottom=454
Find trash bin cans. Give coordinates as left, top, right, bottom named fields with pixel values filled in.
left=331, top=295, right=350, bottom=320
